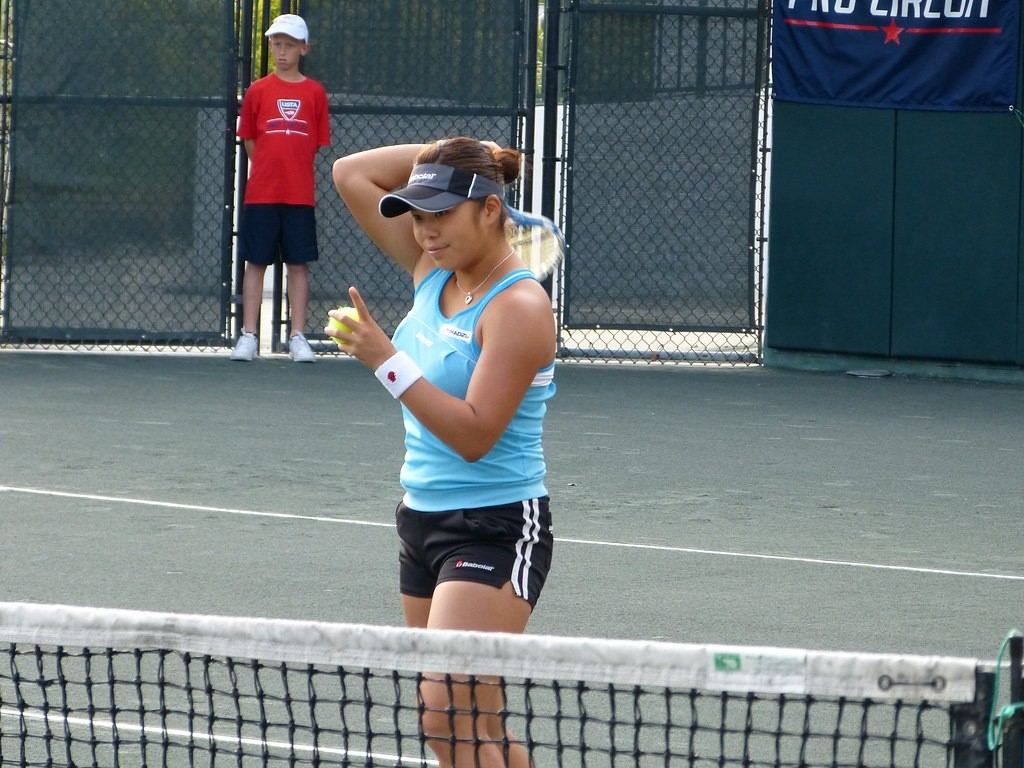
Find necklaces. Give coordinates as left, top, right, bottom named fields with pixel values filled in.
left=456, top=248, right=514, bottom=305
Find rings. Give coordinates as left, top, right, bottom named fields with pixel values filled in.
left=492, top=149, right=498, bottom=155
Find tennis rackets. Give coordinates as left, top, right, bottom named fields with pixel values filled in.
left=502, top=199, right=568, bottom=282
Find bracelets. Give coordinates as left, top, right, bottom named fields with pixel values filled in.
left=374, top=351, right=423, bottom=399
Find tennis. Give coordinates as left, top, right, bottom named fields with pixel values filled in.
left=326, top=306, right=359, bottom=344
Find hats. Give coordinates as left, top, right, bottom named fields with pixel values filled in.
left=265, top=14, right=308, bottom=44
left=378, top=162, right=505, bottom=218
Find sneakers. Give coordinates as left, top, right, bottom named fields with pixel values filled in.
left=289, top=332, right=316, bottom=363
left=230, top=328, right=258, bottom=361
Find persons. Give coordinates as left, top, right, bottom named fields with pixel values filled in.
left=230, top=14, right=332, bottom=362
left=325, top=138, right=555, bottom=768
